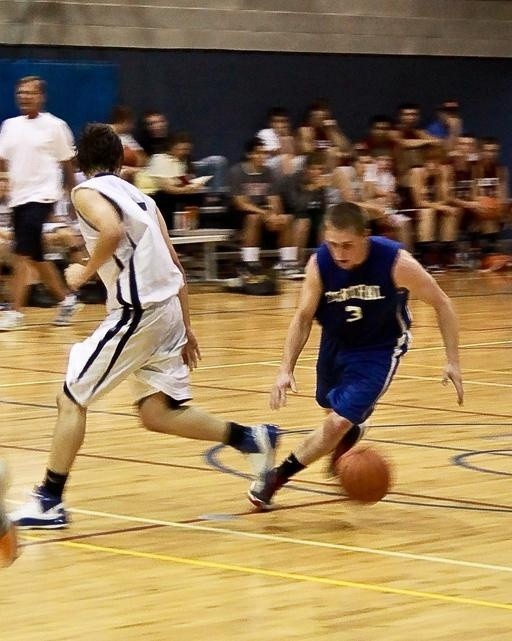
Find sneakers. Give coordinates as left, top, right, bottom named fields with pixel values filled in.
left=6, top=484, right=68, bottom=530
left=51, top=293, right=86, bottom=325
left=321, top=422, right=365, bottom=480
left=246, top=424, right=279, bottom=479
left=248, top=467, right=289, bottom=510
left=420, top=253, right=471, bottom=274
left=239, top=263, right=270, bottom=283
left=0, top=310, right=26, bottom=330
left=273, top=262, right=306, bottom=278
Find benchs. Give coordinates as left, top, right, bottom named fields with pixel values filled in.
left=170, top=204, right=322, bottom=291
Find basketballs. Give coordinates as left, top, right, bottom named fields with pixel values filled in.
left=337, top=447, right=391, bottom=507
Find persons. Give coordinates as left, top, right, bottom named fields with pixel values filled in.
left=0, top=482, right=20, bottom=570
left=244, top=200, right=465, bottom=511
left=0, top=123, right=280, bottom=531
